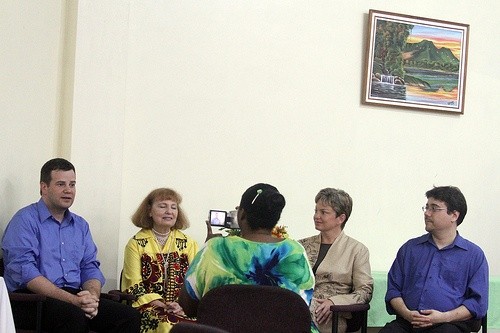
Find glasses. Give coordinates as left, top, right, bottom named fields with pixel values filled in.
left=422, top=205, right=452, bottom=214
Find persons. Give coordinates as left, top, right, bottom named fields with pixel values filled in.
left=121, top=187, right=199, bottom=333
left=2, top=158, right=141, bottom=332
left=177, top=183, right=320, bottom=332
left=211, top=212, right=222, bottom=225
left=380, top=184, right=489, bottom=333
left=298, top=187, right=374, bottom=332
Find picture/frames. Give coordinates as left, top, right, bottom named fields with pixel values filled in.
left=361, top=8, right=470, bottom=116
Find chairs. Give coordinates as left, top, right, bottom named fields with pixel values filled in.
left=8, top=267, right=312, bottom=333
left=329, top=304, right=371, bottom=333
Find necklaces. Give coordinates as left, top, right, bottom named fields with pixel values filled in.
left=153, top=233, right=174, bottom=287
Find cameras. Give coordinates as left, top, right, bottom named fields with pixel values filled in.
left=209, top=210, right=240, bottom=229
